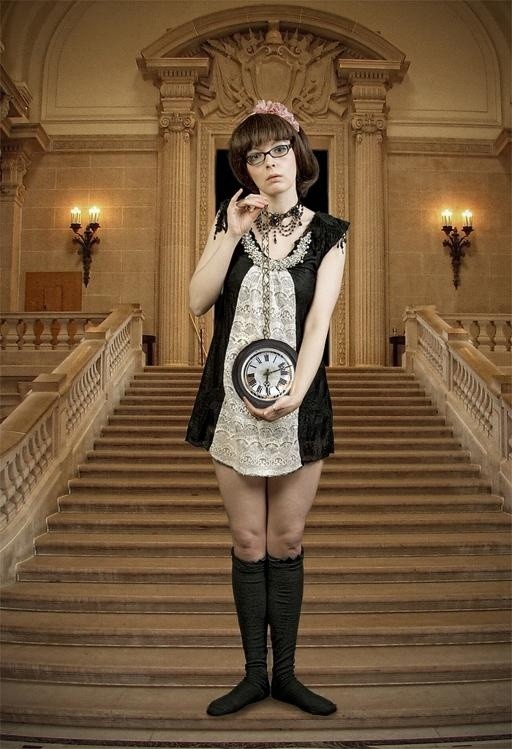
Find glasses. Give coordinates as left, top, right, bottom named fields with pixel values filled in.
left=243, top=142, right=295, bottom=167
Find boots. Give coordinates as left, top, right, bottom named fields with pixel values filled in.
left=203, top=544, right=340, bottom=718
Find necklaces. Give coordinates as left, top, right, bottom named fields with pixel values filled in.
left=250, top=201, right=305, bottom=247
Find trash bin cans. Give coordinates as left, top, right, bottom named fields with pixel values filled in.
left=142, top=335, right=157, bottom=366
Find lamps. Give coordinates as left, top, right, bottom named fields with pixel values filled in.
left=69, top=203, right=102, bottom=290
left=441, top=205, right=476, bottom=292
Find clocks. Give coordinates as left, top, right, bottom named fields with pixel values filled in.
left=233, top=337, right=299, bottom=407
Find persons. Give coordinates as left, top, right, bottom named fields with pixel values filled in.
left=180, top=100, right=353, bottom=720
